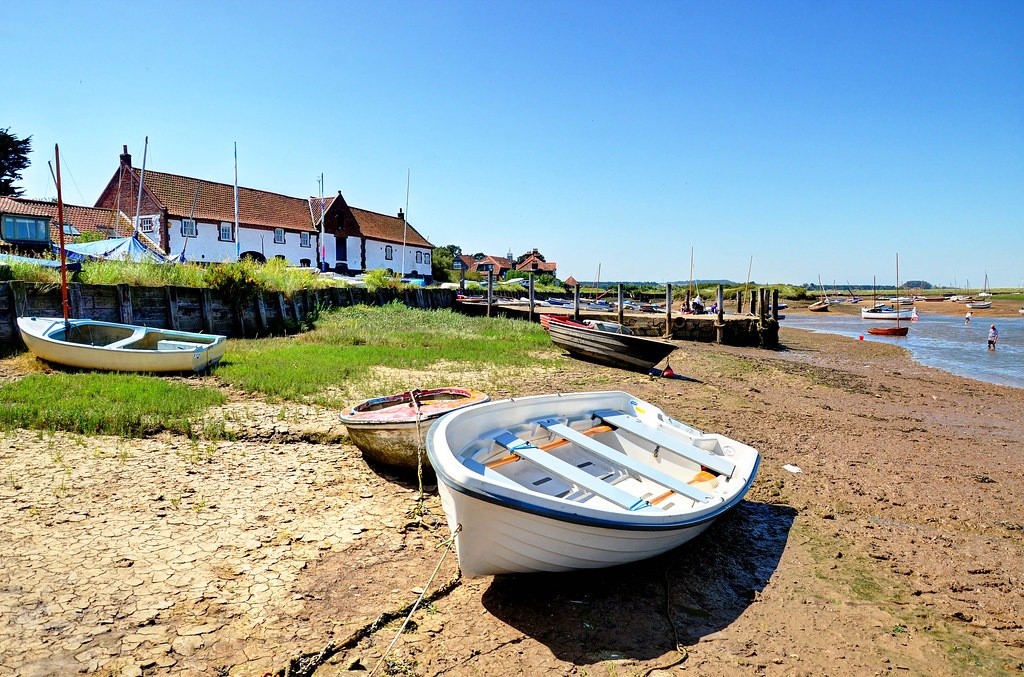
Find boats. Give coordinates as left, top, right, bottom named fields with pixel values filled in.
left=548, top=320, right=680, bottom=372
left=425, top=387, right=763, bottom=580
left=337, top=385, right=491, bottom=473
left=453, top=247, right=788, bottom=336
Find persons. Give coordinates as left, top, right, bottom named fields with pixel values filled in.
left=605, top=300, right=613, bottom=304
left=987, top=325, right=998, bottom=349
left=711, top=302, right=718, bottom=314
left=682, top=303, right=687, bottom=311
left=965, top=312, right=973, bottom=324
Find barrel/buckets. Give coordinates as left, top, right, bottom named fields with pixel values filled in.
left=859, top=336, right=863, bottom=340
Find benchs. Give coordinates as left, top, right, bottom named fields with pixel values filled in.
left=595, top=409, right=736, bottom=477
left=103, top=329, right=148, bottom=348
left=538, top=417, right=715, bottom=503
left=497, top=437, right=667, bottom=513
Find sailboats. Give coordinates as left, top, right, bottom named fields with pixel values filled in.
left=14, top=143, right=229, bottom=376
left=817, top=271, right=994, bottom=321
left=808, top=273, right=830, bottom=312
left=866, top=251, right=908, bottom=337
